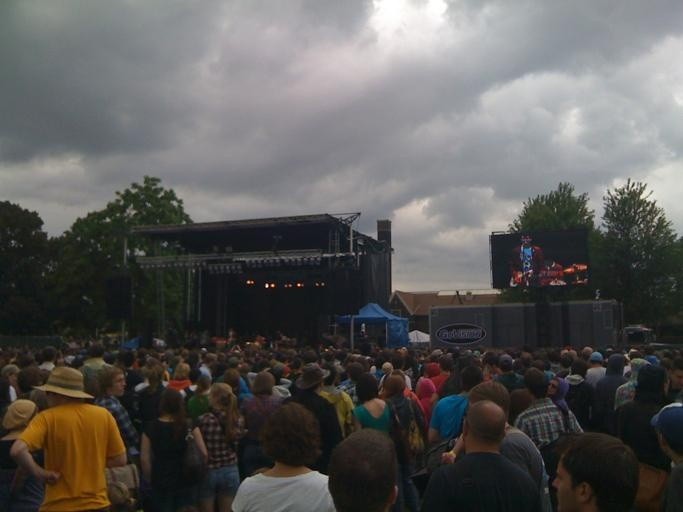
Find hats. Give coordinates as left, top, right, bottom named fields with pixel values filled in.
left=589, top=353, right=603, bottom=362
left=499, top=354, right=511, bottom=369
left=1, top=399, right=35, bottom=431
left=33, top=366, right=93, bottom=399
left=648, top=401, right=682, bottom=452
left=294, top=362, right=330, bottom=390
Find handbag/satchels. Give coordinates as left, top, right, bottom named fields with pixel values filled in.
left=104, top=465, right=140, bottom=491
left=408, top=421, right=424, bottom=457
left=185, top=428, right=208, bottom=474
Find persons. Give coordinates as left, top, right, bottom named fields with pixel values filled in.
left=1, top=328, right=682, bottom=511
left=510, top=232, right=543, bottom=288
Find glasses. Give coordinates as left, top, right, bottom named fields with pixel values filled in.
left=547, top=382, right=554, bottom=391
